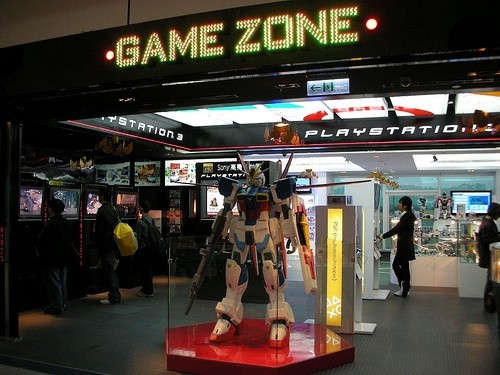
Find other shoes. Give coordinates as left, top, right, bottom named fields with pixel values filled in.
left=137, top=289, right=155, bottom=297
left=394, top=290, right=410, bottom=296
left=401, top=281, right=410, bottom=297
left=100, top=299, right=117, bottom=304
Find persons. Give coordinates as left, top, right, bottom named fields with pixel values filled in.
left=478, top=203, right=500, bottom=314
left=40, top=199, right=74, bottom=314
left=94, top=188, right=121, bottom=304
left=377, top=196, right=418, bottom=296
left=184, top=164, right=318, bottom=350
left=436, top=192, right=454, bottom=219
left=135, top=200, right=156, bottom=297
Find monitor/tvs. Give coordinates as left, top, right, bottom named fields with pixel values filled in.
left=295, top=175, right=312, bottom=194
left=450, top=190, right=492, bottom=216
left=200, top=185, right=240, bottom=221
left=18, top=186, right=139, bottom=222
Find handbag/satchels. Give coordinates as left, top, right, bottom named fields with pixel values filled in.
left=113, top=222, right=138, bottom=256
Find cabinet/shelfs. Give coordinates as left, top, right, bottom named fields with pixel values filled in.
left=389, top=217, right=487, bottom=298
